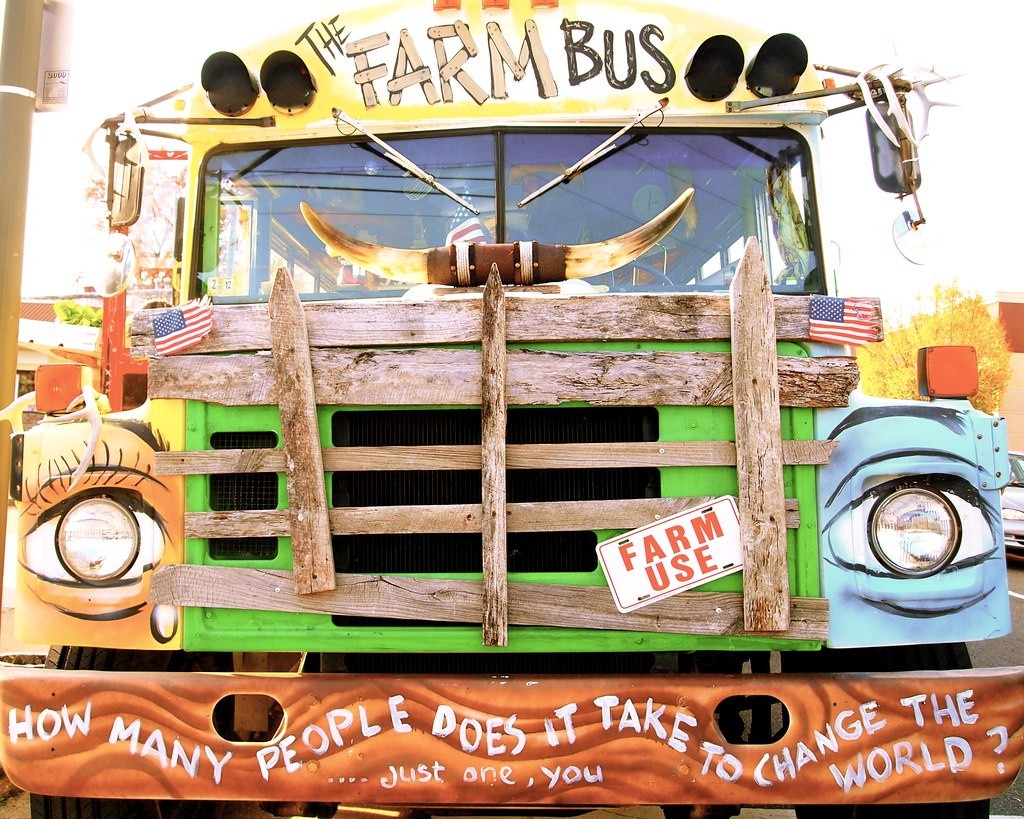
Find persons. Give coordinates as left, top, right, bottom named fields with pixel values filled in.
left=509, top=163, right=589, bottom=244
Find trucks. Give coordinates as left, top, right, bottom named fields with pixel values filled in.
left=0, top=0, right=1024, bottom=818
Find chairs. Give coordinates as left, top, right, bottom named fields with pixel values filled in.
left=582, top=261, right=674, bottom=287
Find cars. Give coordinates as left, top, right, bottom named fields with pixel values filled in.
left=1000, top=450, right=1023, bottom=566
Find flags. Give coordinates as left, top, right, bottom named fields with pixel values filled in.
left=809, top=294, right=879, bottom=348
left=445, top=182, right=493, bottom=244
left=151, top=294, right=213, bottom=356
